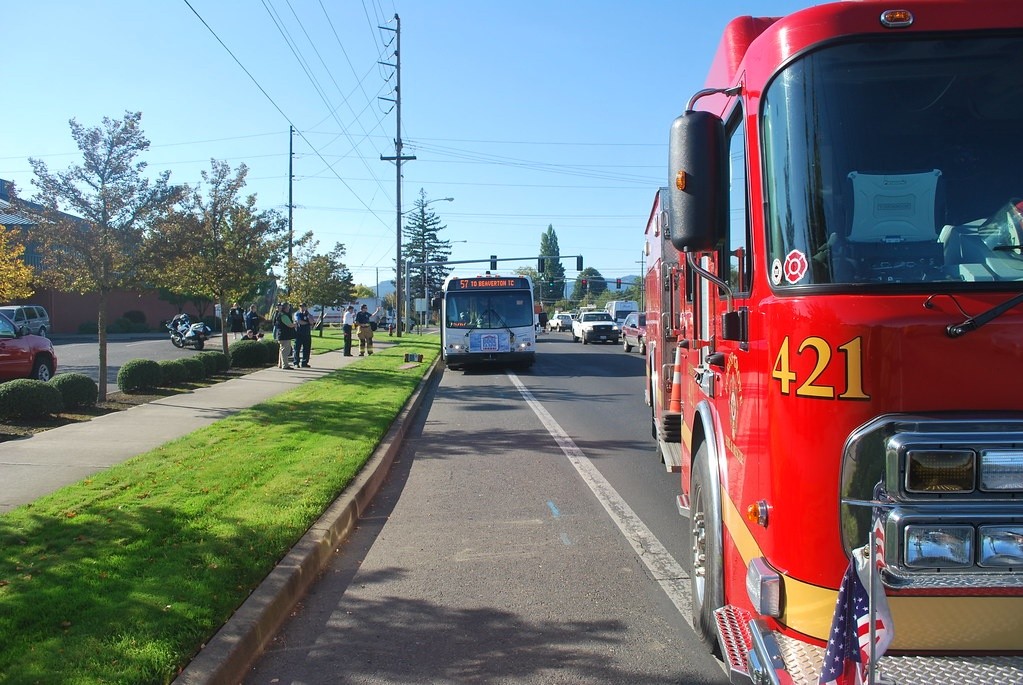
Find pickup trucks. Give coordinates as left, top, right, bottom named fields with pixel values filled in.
left=548, top=311, right=618, bottom=345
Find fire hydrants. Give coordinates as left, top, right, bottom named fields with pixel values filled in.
left=388, top=324, right=392, bottom=336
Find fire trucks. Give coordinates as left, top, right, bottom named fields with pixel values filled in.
left=642, top=0, right=1023, bottom=685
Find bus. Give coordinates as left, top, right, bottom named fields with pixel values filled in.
left=441, top=269, right=536, bottom=371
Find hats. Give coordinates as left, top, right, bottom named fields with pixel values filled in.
left=299, top=302, right=307, bottom=307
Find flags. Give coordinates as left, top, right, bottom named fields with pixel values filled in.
left=870, top=481, right=889, bottom=566
left=816, top=544, right=894, bottom=685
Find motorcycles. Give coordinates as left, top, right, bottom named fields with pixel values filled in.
left=165, top=312, right=212, bottom=350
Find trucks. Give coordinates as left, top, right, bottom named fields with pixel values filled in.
left=604, top=299, right=638, bottom=333
left=293, top=298, right=381, bottom=331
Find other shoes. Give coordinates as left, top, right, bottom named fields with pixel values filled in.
left=301, top=363, right=310, bottom=367
left=359, top=353, right=364, bottom=356
left=294, top=364, right=300, bottom=369
left=284, top=366, right=294, bottom=370
left=346, top=354, right=353, bottom=356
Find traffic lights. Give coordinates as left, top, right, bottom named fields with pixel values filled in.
left=617, top=279, right=621, bottom=289
left=549, top=276, right=553, bottom=287
left=582, top=279, right=587, bottom=289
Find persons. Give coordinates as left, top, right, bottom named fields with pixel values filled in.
left=343, top=306, right=354, bottom=356
left=272, top=302, right=297, bottom=370
left=355, top=304, right=381, bottom=356
left=240, top=330, right=264, bottom=341
left=230, top=303, right=245, bottom=340
left=293, top=303, right=314, bottom=368
left=246, top=304, right=259, bottom=334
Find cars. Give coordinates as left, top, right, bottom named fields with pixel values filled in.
left=0, top=313, right=57, bottom=384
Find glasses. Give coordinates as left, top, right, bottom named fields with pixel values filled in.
left=364, top=307, right=367, bottom=309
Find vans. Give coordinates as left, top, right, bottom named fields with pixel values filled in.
left=0, top=306, right=51, bottom=339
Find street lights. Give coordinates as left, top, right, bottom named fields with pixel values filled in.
left=425, top=240, right=467, bottom=328
left=396, top=198, right=454, bottom=337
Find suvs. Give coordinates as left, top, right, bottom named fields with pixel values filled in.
left=622, top=312, right=646, bottom=355
